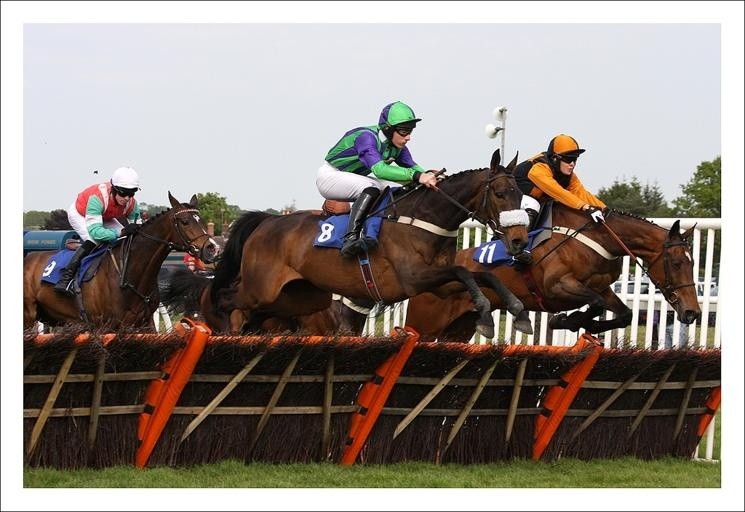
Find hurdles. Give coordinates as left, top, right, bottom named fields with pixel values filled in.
left=338, top=332, right=597, bottom=467
left=529, top=344, right=721, bottom=461
left=23, top=319, right=196, bottom=471
left=135, top=321, right=407, bottom=467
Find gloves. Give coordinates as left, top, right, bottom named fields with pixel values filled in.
left=581, top=204, right=606, bottom=223
left=121, top=223, right=138, bottom=236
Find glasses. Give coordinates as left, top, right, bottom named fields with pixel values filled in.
left=110, top=179, right=134, bottom=198
left=389, top=125, right=413, bottom=137
left=557, top=154, right=577, bottom=163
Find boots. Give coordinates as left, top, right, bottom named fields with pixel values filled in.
left=52, top=239, right=97, bottom=297
left=508, top=208, right=540, bottom=265
left=339, top=187, right=380, bottom=258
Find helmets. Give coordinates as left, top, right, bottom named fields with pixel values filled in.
left=547, top=134, right=586, bottom=161
left=109, top=167, right=140, bottom=189
left=378, top=101, right=422, bottom=130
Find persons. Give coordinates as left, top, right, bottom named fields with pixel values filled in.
left=510, top=134, right=608, bottom=266
left=315, top=101, right=438, bottom=258
left=53, top=166, right=144, bottom=298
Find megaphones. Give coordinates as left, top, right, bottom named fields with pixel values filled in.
left=492, top=106, right=508, bottom=121
left=486, top=124, right=500, bottom=139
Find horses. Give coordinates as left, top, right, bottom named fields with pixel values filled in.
left=157, top=148, right=533, bottom=340
left=404, top=199, right=702, bottom=341
left=23, top=190, right=221, bottom=335
